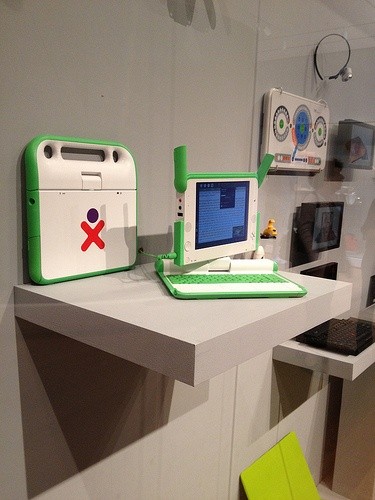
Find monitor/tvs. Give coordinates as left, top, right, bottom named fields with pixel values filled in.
left=173, top=173, right=259, bottom=266
left=295, top=202, right=344, bottom=254
left=334, top=118, right=375, bottom=171
left=300, top=262, right=337, bottom=280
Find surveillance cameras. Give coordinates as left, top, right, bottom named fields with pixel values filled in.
left=338, top=67, right=353, bottom=82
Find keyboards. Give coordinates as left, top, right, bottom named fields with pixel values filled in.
left=153, top=258, right=308, bottom=300
left=295, top=316, right=375, bottom=356
